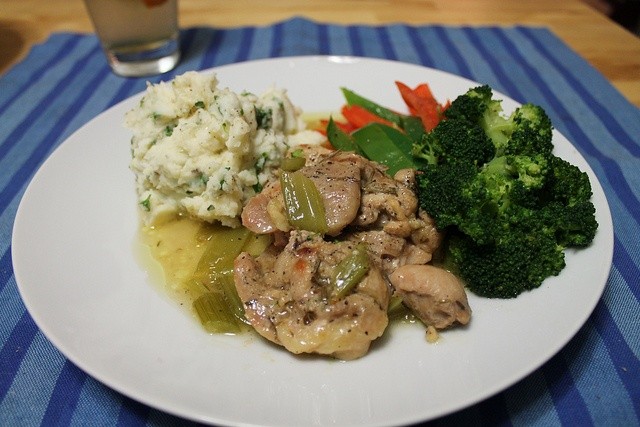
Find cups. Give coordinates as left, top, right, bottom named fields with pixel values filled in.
left=81, top=0, right=182, bottom=78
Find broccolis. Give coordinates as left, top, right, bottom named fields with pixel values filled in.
left=408, top=84, right=599, bottom=298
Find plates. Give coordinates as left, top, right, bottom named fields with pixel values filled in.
left=8, top=51, right=616, bottom=427
